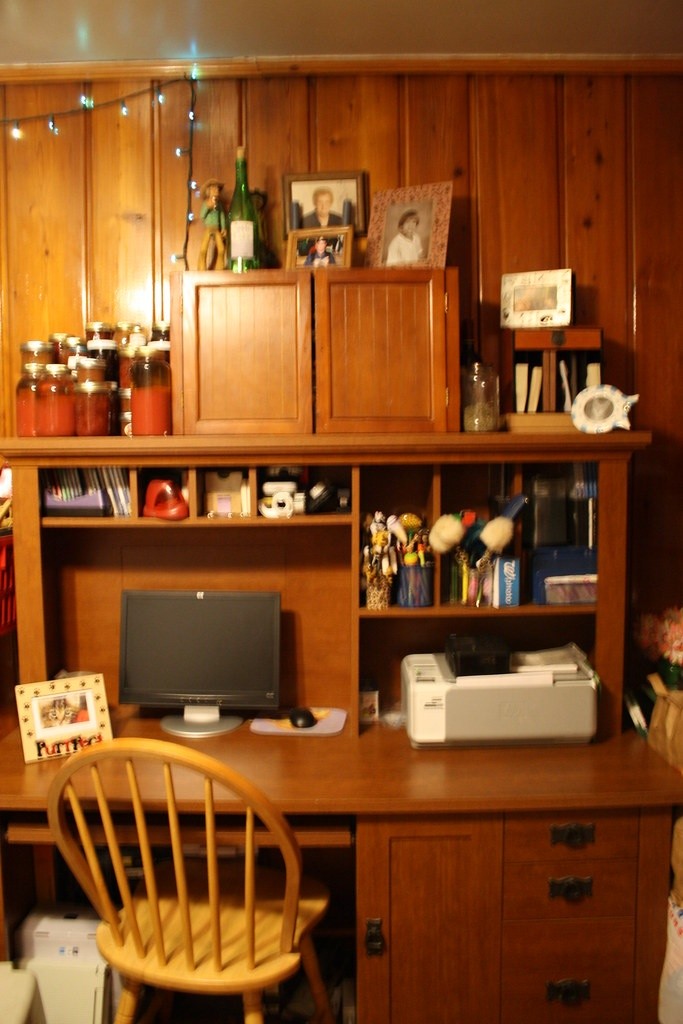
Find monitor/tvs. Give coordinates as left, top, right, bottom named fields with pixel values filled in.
left=118, top=589, right=280, bottom=739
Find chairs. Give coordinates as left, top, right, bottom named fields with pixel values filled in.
left=48, top=738, right=338, bottom=1024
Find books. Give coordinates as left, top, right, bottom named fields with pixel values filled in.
left=44, top=466, right=131, bottom=516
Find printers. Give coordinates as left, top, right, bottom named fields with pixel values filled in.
left=401, top=641, right=601, bottom=749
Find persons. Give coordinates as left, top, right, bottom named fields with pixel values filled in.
left=198, top=178, right=226, bottom=269
left=304, top=235, right=336, bottom=265
left=300, top=185, right=345, bottom=231
left=385, top=208, right=424, bottom=265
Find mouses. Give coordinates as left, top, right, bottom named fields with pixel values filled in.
left=289, top=706, right=315, bottom=729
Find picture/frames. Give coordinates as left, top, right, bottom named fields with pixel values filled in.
left=15, top=673, right=113, bottom=765
left=286, top=224, right=352, bottom=270
left=281, top=168, right=367, bottom=240
left=571, top=384, right=639, bottom=434
left=501, top=268, right=571, bottom=327
left=364, top=180, right=453, bottom=269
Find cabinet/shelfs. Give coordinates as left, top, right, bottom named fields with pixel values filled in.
left=0, top=270, right=683, bottom=1024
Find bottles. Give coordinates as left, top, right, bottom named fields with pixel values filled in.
left=228, top=147, right=261, bottom=269
left=463, top=361, right=501, bottom=434
left=16, top=321, right=173, bottom=439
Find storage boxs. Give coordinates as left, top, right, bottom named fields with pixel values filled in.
left=13, top=902, right=123, bottom=1021
